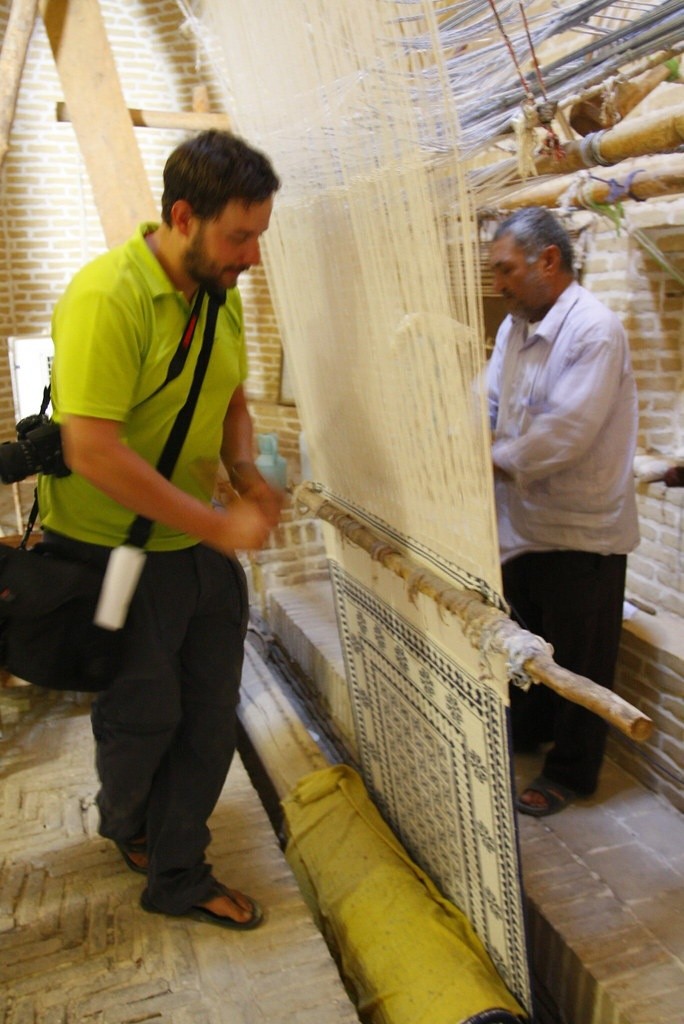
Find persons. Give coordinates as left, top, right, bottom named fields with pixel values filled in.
left=470, top=201, right=642, bottom=817
left=37, top=130, right=285, bottom=927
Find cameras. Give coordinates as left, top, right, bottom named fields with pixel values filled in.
left=0, top=413, right=73, bottom=485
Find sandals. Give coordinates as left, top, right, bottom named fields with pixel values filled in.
left=514, top=776, right=574, bottom=816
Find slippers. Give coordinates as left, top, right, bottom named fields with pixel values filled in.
left=138, top=882, right=262, bottom=930
left=113, top=837, right=151, bottom=874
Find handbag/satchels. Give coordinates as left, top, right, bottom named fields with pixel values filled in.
left=0, top=543, right=122, bottom=692
